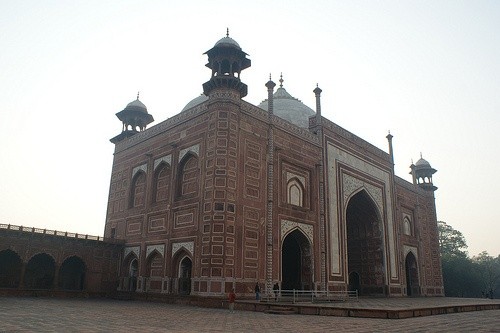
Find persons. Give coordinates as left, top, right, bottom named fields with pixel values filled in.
left=254, top=284, right=262, bottom=303
left=272, top=281, right=280, bottom=300
left=490, top=288, right=494, bottom=299
left=227, top=286, right=237, bottom=315
left=482, top=289, right=485, bottom=299
left=485, top=290, right=489, bottom=300
